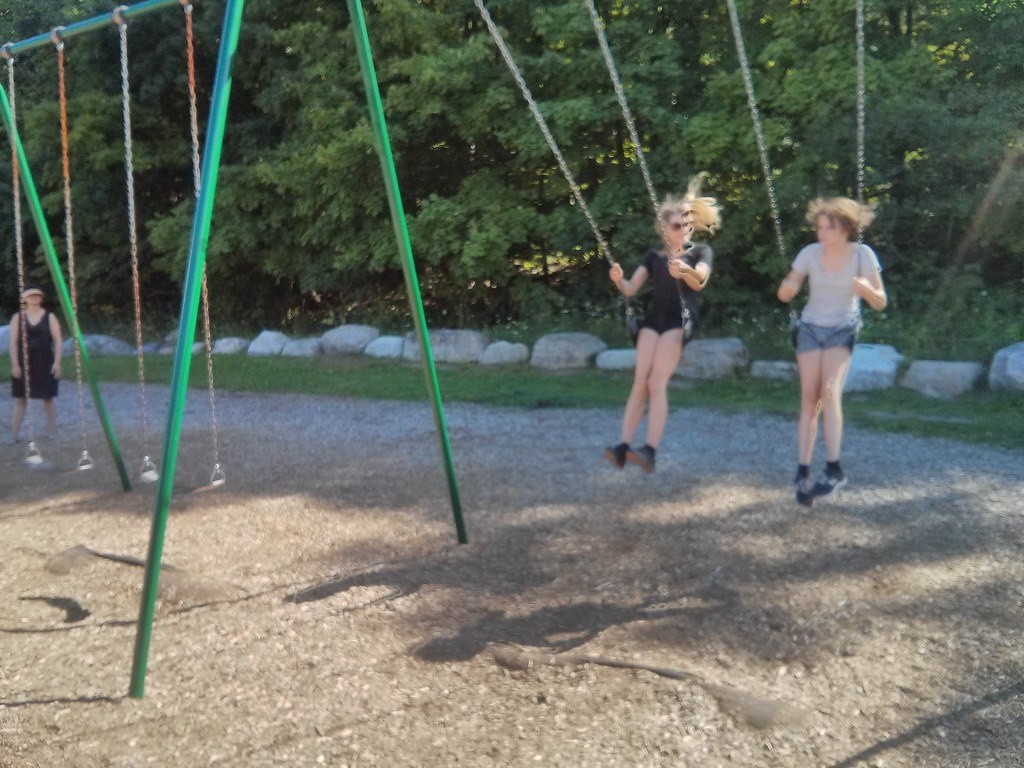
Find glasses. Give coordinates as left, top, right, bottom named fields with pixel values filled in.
left=671, top=221, right=693, bottom=232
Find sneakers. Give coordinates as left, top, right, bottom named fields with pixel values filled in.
left=794, top=471, right=814, bottom=508
left=810, top=468, right=848, bottom=499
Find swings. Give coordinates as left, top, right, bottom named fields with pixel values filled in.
left=2, top=43, right=102, bottom=483
left=459, top=0, right=699, bottom=355
left=720, top=0, right=875, bottom=361
left=106, top=5, right=240, bottom=497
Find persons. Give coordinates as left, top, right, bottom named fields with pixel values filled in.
left=603, top=198, right=721, bottom=473
left=8, top=285, right=62, bottom=445
left=777, top=196, right=887, bottom=507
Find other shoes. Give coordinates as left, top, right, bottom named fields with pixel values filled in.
left=8, top=437, right=17, bottom=445
left=48, top=433, right=57, bottom=439
left=604, top=442, right=630, bottom=469
left=626, top=444, right=655, bottom=474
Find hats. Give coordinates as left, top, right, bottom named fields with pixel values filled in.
left=22, top=284, right=45, bottom=298
